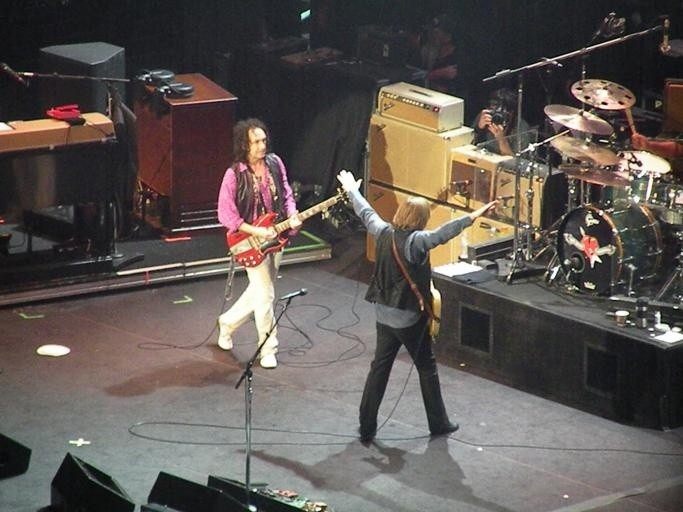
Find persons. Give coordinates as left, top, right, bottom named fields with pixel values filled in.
left=468, top=84, right=531, bottom=162
left=337, top=168, right=501, bottom=439
left=425, top=23, right=469, bottom=101
left=216, top=117, right=302, bottom=368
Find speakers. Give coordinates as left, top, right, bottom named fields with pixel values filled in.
left=1, top=433, right=32, bottom=479
left=24, top=39, right=128, bottom=239
left=49, top=451, right=135, bottom=512
left=207, top=473, right=329, bottom=512
left=141, top=469, right=252, bottom=512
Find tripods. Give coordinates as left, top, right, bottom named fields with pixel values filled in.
left=481, top=25, right=662, bottom=285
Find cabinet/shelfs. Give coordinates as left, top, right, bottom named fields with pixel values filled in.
left=135, top=75, right=239, bottom=238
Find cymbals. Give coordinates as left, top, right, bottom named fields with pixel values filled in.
left=556, top=164, right=633, bottom=189
left=548, top=136, right=619, bottom=165
left=542, top=104, right=614, bottom=136
left=571, top=79, right=637, bottom=110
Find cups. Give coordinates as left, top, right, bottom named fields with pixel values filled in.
left=615, top=310, right=630, bottom=328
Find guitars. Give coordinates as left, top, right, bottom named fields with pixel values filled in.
left=428, top=281, right=442, bottom=338
left=225, top=187, right=350, bottom=268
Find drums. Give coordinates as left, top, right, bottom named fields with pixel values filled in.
left=555, top=199, right=662, bottom=295
left=657, top=184, right=682, bottom=223
left=628, top=168, right=670, bottom=212
left=602, top=149, right=671, bottom=203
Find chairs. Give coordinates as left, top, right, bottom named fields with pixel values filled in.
left=654, top=78, right=681, bottom=145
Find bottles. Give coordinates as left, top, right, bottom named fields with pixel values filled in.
left=655, top=311, right=661, bottom=324
left=636, top=299, right=648, bottom=329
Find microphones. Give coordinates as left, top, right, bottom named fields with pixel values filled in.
left=279, top=287, right=309, bottom=302
left=658, top=18, right=671, bottom=56
left=1, top=64, right=28, bottom=88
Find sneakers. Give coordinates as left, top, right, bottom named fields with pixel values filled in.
left=258, top=350, right=278, bottom=369
left=426, top=420, right=460, bottom=435
left=216, top=313, right=233, bottom=349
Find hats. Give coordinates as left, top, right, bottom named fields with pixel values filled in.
left=421, top=14, right=455, bottom=31
